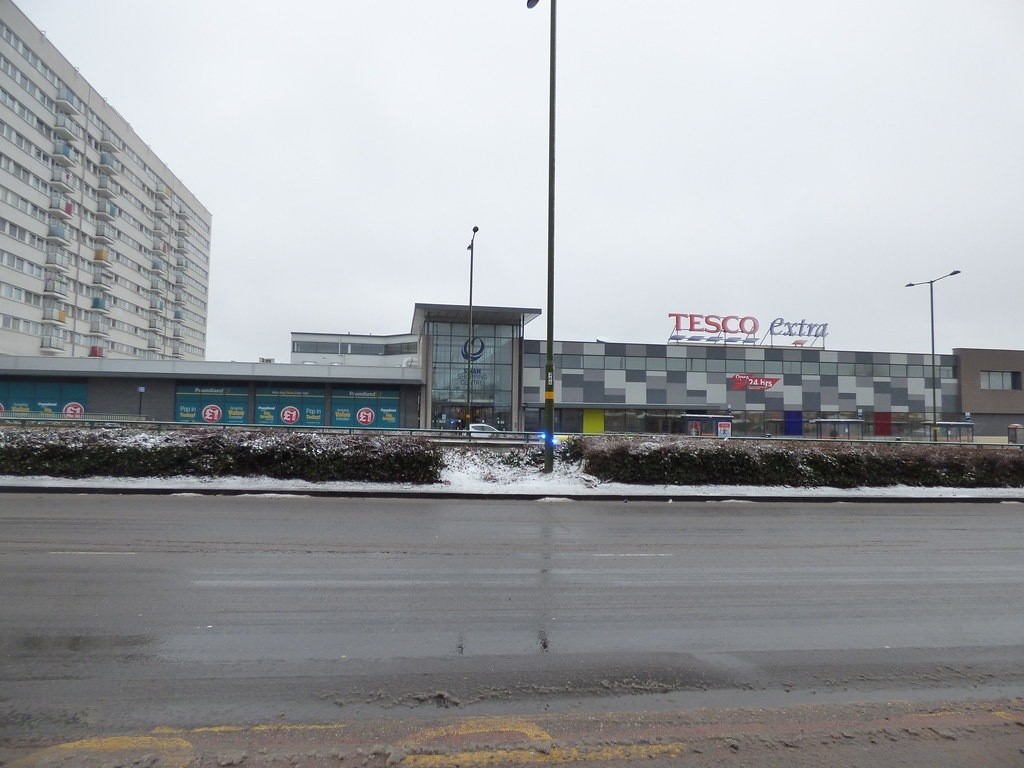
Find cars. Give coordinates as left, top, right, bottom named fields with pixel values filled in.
left=461, top=424, right=503, bottom=438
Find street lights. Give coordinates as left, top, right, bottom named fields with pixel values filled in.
left=466, top=227, right=478, bottom=437
left=527, top=0, right=555, bottom=475
left=904, top=271, right=962, bottom=442
